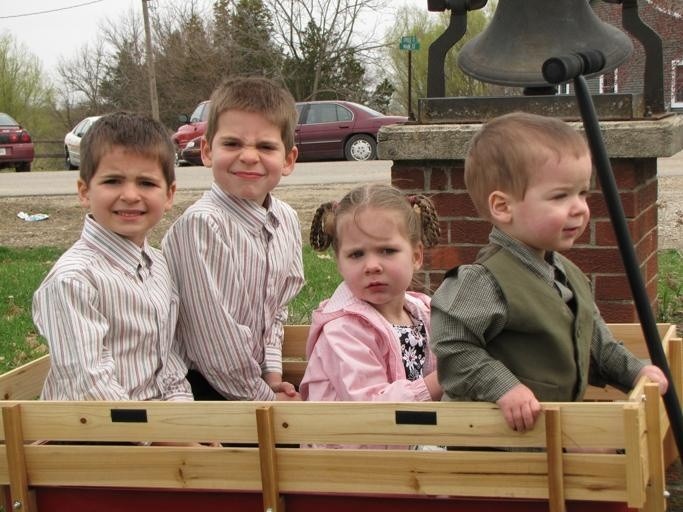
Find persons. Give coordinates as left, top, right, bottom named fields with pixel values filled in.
left=27, top=109, right=224, bottom=449
left=427, top=109, right=670, bottom=457
left=160, top=76, right=306, bottom=402
left=294, top=182, right=458, bottom=453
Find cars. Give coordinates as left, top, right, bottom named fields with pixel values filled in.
left=172, top=99, right=409, bottom=167
left=0, top=111, right=34, bottom=172
left=64, top=113, right=103, bottom=170
